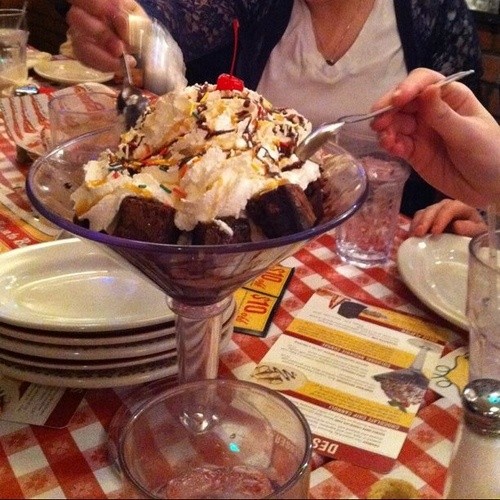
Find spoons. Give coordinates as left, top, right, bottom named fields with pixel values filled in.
left=116, top=40, right=148, bottom=131
left=293, top=68, right=475, bottom=162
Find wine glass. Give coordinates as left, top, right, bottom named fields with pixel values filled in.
left=23, top=124, right=369, bottom=486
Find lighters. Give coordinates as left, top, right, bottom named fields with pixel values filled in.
left=15, top=87, right=60, bottom=96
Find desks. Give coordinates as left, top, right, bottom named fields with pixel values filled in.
left=1, top=43, right=467, bottom=500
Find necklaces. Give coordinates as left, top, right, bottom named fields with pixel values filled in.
left=310, top=0, right=362, bottom=65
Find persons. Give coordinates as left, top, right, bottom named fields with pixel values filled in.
left=370, top=67, right=500, bottom=238
left=67, top=0, right=484, bottom=233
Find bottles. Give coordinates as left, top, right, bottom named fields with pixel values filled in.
left=441, top=377, right=500, bottom=499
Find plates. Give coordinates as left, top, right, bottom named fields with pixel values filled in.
left=0, top=80, right=119, bottom=165
left=397, top=233, right=500, bottom=333
left=33, top=59, right=115, bottom=86
left=0, top=237, right=238, bottom=389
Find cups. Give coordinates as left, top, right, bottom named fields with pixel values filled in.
left=333, top=114, right=410, bottom=265
left=117, top=378, right=313, bottom=500
left=46, top=92, right=121, bottom=162
left=466, top=228, right=500, bottom=439
left=0, top=7, right=30, bottom=98
